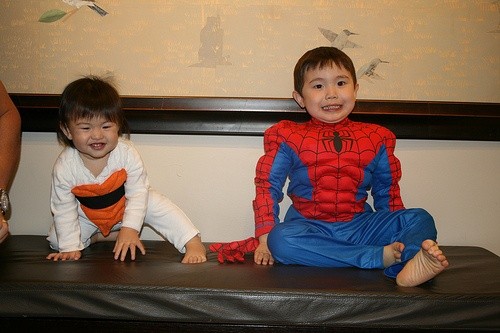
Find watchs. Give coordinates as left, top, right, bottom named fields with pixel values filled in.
left=0, top=189, right=9, bottom=216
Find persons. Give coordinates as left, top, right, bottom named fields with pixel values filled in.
left=252, top=46, right=448, bottom=288
left=45, top=78, right=207, bottom=264
left=0, top=80, right=22, bottom=245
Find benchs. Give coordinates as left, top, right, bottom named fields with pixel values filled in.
left=0, top=235, right=499, bottom=333
